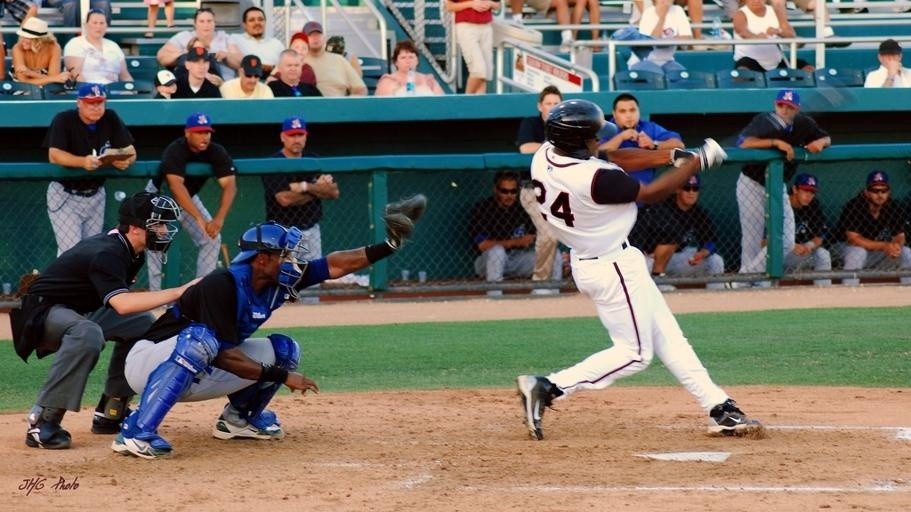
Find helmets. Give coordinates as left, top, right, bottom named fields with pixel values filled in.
left=544, top=98, right=605, bottom=160
left=230, top=221, right=309, bottom=292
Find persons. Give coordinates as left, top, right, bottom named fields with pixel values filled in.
left=627, top=168, right=733, bottom=291
left=517, top=98, right=767, bottom=439
left=0, top=0, right=444, bottom=99
left=519, top=85, right=562, bottom=295
left=593, top=92, right=684, bottom=291
left=762, top=173, right=832, bottom=288
left=110, top=194, right=427, bottom=457
left=25, top=191, right=205, bottom=448
left=445, top=0, right=911, bottom=95
left=829, top=171, right=911, bottom=284
left=46, top=83, right=138, bottom=259
left=724, top=90, right=832, bottom=288
left=469, top=165, right=562, bottom=295
left=259, top=118, right=339, bottom=304
left=143, top=113, right=238, bottom=291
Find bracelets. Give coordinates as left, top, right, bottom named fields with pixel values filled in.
left=259, top=363, right=288, bottom=384
left=365, top=243, right=394, bottom=264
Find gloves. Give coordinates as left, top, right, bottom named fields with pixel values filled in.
left=672, top=137, right=727, bottom=173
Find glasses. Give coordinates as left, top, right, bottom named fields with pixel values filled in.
left=499, top=187, right=517, bottom=195
left=243, top=73, right=260, bottom=79
left=681, top=181, right=699, bottom=192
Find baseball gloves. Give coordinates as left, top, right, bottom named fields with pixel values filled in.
left=384, top=197, right=426, bottom=238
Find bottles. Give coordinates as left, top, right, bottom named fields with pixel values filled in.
left=405, top=68, right=416, bottom=97
left=713, top=14, right=722, bottom=41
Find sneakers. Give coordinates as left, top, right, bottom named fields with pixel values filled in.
left=109, top=423, right=172, bottom=459
left=513, top=375, right=553, bottom=440
left=24, top=414, right=70, bottom=449
left=90, top=401, right=137, bottom=434
left=212, top=404, right=281, bottom=440
left=707, top=401, right=762, bottom=436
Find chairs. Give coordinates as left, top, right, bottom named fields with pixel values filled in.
left=613, top=70, right=665, bottom=92
left=767, top=67, right=814, bottom=88
left=1, top=81, right=43, bottom=101
left=125, top=56, right=160, bottom=80
left=814, top=67, right=866, bottom=89
left=665, top=71, right=717, bottom=89
left=103, top=80, right=156, bottom=98
left=43, top=82, right=95, bottom=101
left=718, top=69, right=766, bottom=90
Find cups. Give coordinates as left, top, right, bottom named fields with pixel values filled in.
left=402, top=271, right=410, bottom=280
left=3, top=283, right=11, bottom=295
left=419, top=272, right=427, bottom=283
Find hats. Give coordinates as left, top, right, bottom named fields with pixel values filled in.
left=185, top=46, right=211, bottom=63
left=304, top=21, right=323, bottom=35
left=279, top=116, right=309, bottom=137
left=152, top=69, right=177, bottom=87
left=792, top=172, right=820, bottom=193
left=774, top=89, right=801, bottom=110
left=865, top=170, right=890, bottom=188
left=878, top=39, right=904, bottom=56
left=14, top=17, right=50, bottom=40
left=240, top=54, right=262, bottom=73
left=184, top=112, right=215, bottom=135
left=76, top=82, right=108, bottom=103
left=289, top=31, right=310, bottom=48
